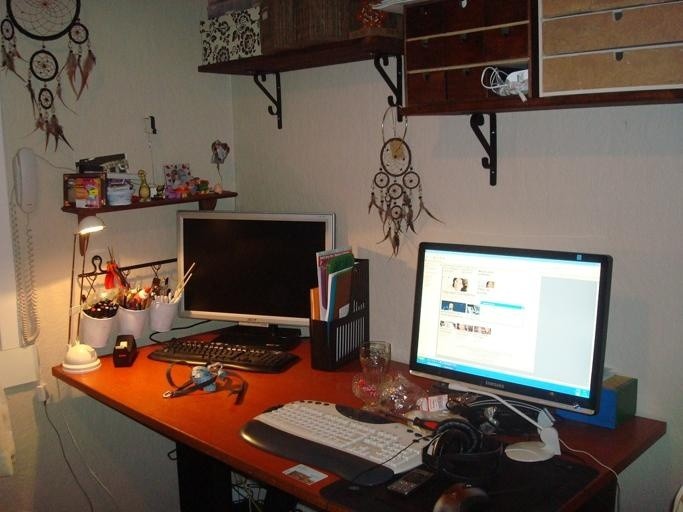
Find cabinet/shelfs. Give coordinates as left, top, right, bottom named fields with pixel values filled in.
left=399, top=0, right=682, bottom=116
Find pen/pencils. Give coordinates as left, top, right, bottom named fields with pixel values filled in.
left=81, top=276, right=174, bottom=319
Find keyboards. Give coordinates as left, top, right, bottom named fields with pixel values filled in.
left=239, top=399, right=434, bottom=487
left=147, top=337, right=299, bottom=373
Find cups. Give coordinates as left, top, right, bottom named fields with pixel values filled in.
left=79, top=307, right=119, bottom=350
left=151, top=300, right=179, bottom=333
left=117, top=305, right=151, bottom=341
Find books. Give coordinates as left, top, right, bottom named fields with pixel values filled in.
left=304, top=244, right=361, bottom=323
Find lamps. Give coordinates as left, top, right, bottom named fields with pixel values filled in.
left=61, top=215, right=105, bottom=374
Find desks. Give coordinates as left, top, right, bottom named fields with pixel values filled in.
left=51, top=326, right=667, bottom=510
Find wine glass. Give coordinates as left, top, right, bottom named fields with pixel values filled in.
left=358, top=339, right=392, bottom=415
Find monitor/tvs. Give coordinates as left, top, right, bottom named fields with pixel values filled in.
left=408, top=241, right=614, bottom=435
left=175, top=210, right=336, bottom=352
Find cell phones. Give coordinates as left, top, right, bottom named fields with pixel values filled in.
left=387, top=468, right=435, bottom=495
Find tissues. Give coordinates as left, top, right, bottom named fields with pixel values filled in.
left=556, top=364, right=637, bottom=431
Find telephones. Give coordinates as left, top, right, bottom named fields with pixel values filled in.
left=12, top=147, right=39, bottom=213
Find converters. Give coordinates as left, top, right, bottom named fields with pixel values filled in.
left=36, top=383, right=49, bottom=402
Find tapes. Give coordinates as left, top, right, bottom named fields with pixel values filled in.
left=120, top=340, right=128, bottom=348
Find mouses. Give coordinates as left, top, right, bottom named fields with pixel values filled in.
left=433, top=483, right=487, bottom=512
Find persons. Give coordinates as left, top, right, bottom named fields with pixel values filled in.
left=439, top=277, right=495, bottom=336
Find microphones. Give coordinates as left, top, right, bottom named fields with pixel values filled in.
left=447, top=383, right=562, bottom=463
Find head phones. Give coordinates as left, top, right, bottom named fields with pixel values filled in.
left=422, top=418, right=505, bottom=481
